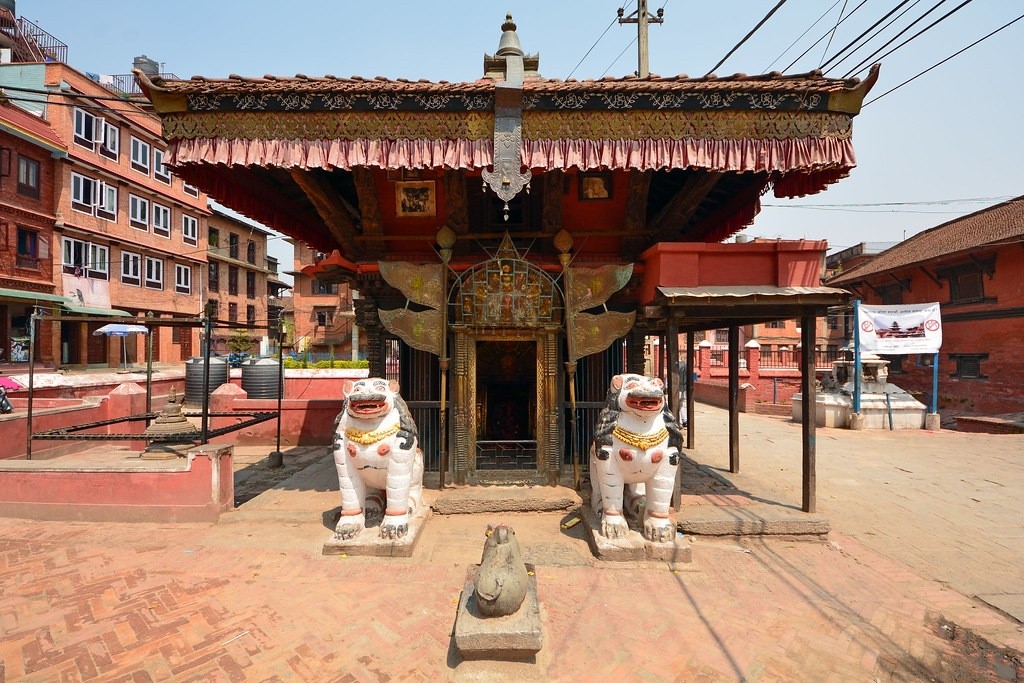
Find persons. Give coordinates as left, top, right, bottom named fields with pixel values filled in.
left=68, top=289, right=84, bottom=306
left=678, top=354, right=687, bottom=428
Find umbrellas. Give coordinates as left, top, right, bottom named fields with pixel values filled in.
left=93, top=324, right=149, bottom=370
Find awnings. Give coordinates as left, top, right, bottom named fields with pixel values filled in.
left=55, top=303, right=132, bottom=317
left=1, top=288, right=73, bottom=302
